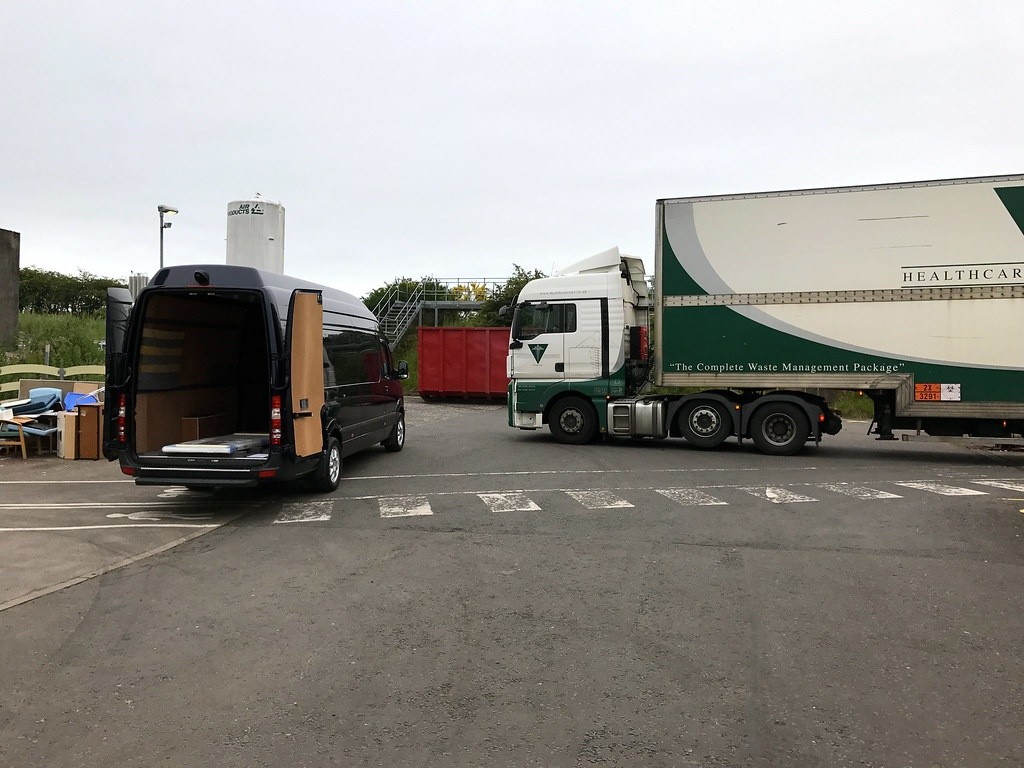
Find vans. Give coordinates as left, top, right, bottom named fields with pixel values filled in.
left=103, top=264, right=410, bottom=492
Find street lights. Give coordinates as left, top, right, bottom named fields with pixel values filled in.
left=157, top=204, right=179, bottom=269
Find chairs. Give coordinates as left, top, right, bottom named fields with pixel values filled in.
left=0, top=418, right=27, bottom=460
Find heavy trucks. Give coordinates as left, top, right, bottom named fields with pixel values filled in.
left=499, top=173, right=1023, bottom=460
